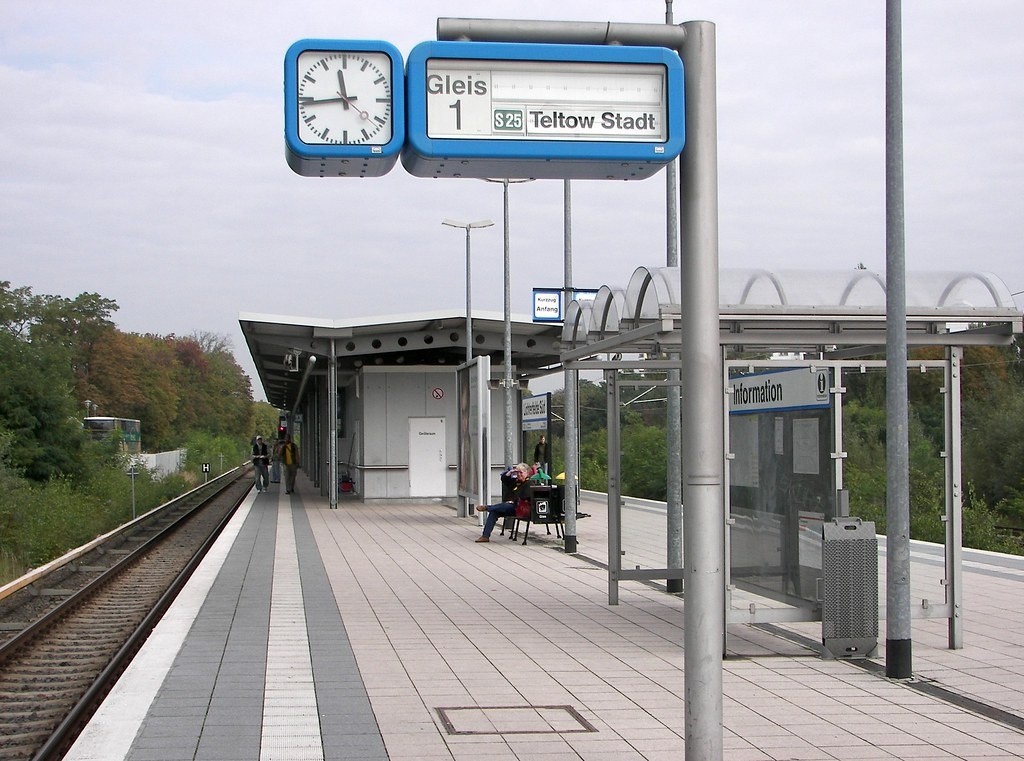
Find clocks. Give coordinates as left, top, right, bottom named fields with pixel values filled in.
left=283, top=37, right=405, bottom=177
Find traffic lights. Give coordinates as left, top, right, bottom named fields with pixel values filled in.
left=278, top=426, right=285, bottom=439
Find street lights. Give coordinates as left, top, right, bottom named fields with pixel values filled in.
left=442, top=217, right=495, bottom=360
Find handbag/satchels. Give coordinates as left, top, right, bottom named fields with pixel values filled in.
left=515, top=498, right=531, bottom=517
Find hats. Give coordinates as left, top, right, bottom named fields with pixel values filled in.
left=256, top=435, right=262, bottom=440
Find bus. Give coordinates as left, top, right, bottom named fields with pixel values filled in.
left=83, top=417, right=141, bottom=457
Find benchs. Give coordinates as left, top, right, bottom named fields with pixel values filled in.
left=500, top=511, right=591, bottom=546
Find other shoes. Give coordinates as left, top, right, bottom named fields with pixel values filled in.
left=475, top=536, right=489, bottom=542
left=291, top=488, right=294, bottom=492
left=476, top=505, right=491, bottom=511
left=262, top=487, right=267, bottom=493
left=257, top=489, right=261, bottom=493
left=285, top=490, right=290, bottom=494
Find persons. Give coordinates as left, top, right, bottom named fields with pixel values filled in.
left=280, top=434, right=302, bottom=494
left=475, top=462, right=532, bottom=541
left=534, top=434, right=549, bottom=473
left=252, top=435, right=269, bottom=494
left=270, top=438, right=281, bottom=484
